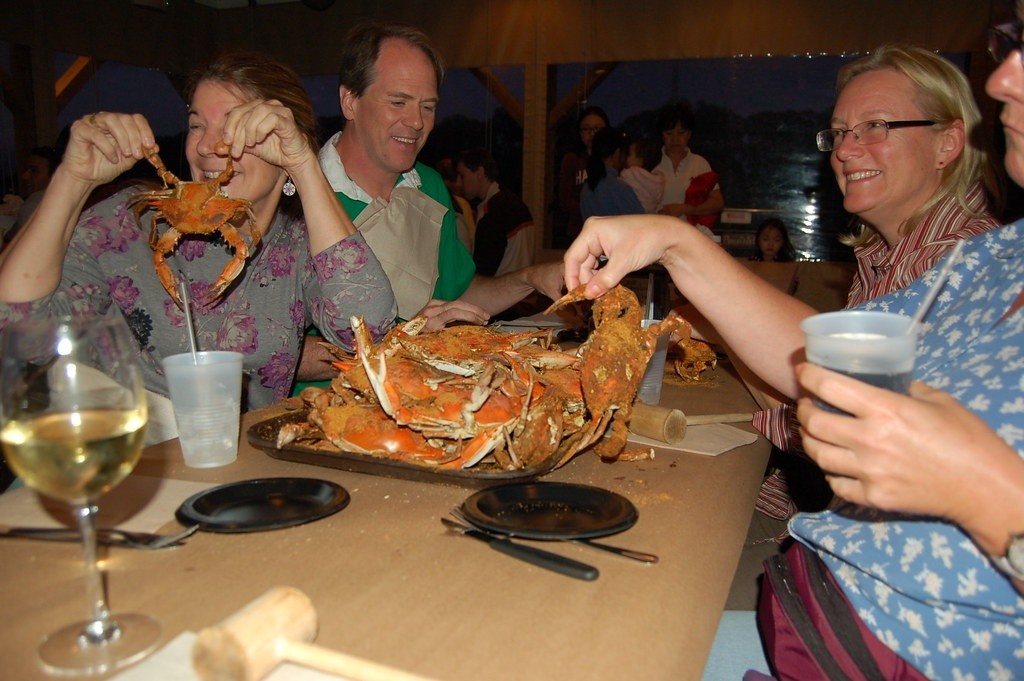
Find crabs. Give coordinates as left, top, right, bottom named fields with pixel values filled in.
left=125, top=141, right=262, bottom=310
left=276, top=280, right=716, bottom=471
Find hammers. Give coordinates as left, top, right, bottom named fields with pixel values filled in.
left=193, top=585, right=434, bottom=680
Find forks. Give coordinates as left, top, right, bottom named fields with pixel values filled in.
left=449, top=504, right=659, bottom=563
left=8, top=523, right=201, bottom=548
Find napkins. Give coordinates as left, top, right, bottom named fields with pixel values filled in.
left=492, top=308, right=570, bottom=335
left=352, top=185, right=449, bottom=321
left=110, top=630, right=345, bottom=681
left=601, top=414, right=760, bottom=456
left=1, top=474, right=219, bottom=534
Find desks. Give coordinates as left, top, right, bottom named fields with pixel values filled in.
left=0, top=330, right=769, bottom=681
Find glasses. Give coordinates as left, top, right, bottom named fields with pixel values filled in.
left=815, top=119, right=938, bottom=151
left=987, top=23, right=1024, bottom=66
left=579, top=126, right=600, bottom=133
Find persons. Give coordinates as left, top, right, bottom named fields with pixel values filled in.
left=563, top=1, right=1024, bottom=681
left=559, top=106, right=609, bottom=242
left=668, top=40, right=1005, bottom=520
left=315, top=20, right=565, bottom=335
left=0, top=48, right=399, bottom=416
left=579, top=127, right=645, bottom=223
left=740, top=218, right=809, bottom=261
left=430, top=152, right=475, bottom=255
left=455, top=142, right=535, bottom=279
left=616, top=137, right=666, bottom=214
left=649, top=97, right=724, bottom=219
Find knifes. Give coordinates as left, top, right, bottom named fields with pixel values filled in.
left=439, top=517, right=598, bottom=582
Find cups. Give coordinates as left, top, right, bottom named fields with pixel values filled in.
left=800, top=310, right=921, bottom=416
left=163, top=351, right=244, bottom=469
left=637, top=319, right=669, bottom=405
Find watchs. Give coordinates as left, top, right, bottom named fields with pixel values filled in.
left=993, top=531, right=1024, bottom=587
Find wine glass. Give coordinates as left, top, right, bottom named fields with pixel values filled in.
left=0, top=311, right=164, bottom=676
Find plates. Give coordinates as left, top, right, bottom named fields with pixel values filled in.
left=175, top=478, right=350, bottom=533
left=460, top=482, right=639, bottom=540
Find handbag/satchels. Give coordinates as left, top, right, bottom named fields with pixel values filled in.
left=757, top=543, right=931, bottom=681
